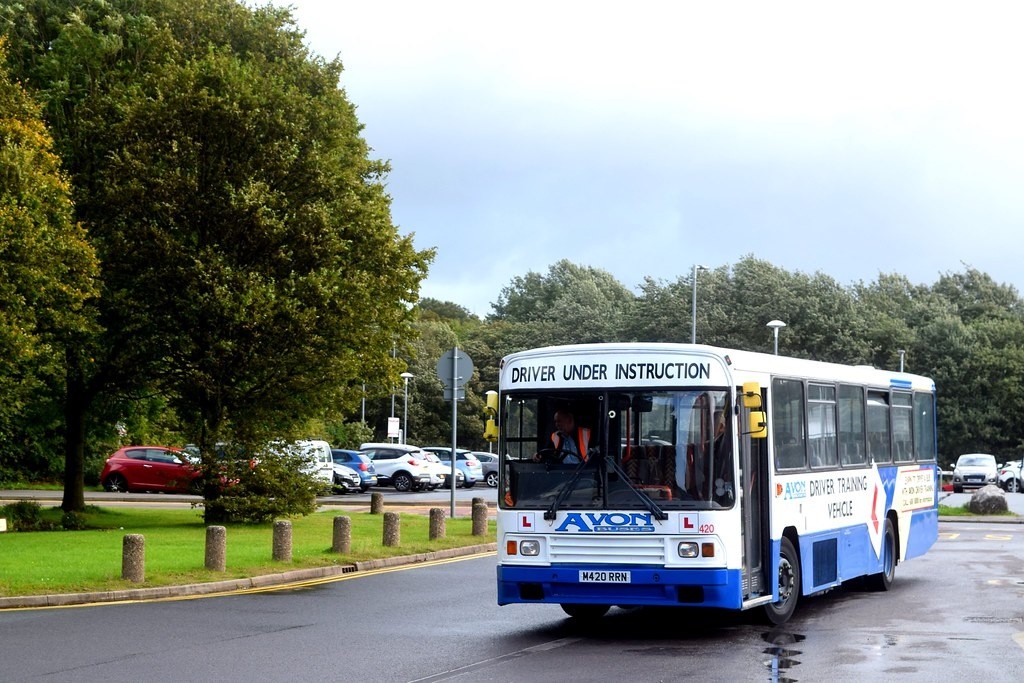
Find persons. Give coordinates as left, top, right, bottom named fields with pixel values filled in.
left=532, top=409, right=591, bottom=464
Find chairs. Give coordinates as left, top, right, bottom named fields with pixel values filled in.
left=779, top=438, right=913, bottom=468
left=619, top=444, right=694, bottom=488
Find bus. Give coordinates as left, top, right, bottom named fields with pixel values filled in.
left=483, top=343, right=938, bottom=625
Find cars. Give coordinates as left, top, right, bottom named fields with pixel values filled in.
left=331, top=449, right=378, bottom=493
left=470, top=451, right=499, bottom=488
left=998, top=459, right=1024, bottom=492
left=950, top=453, right=1003, bottom=493
left=333, top=462, right=362, bottom=495
left=359, top=443, right=484, bottom=492
left=184, top=440, right=334, bottom=496
left=100, top=446, right=241, bottom=496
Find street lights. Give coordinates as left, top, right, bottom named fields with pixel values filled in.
left=400, top=372, right=413, bottom=445
left=391, top=342, right=396, bottom=444
left=898, top=350, right=905, bottom=373
left=766, top=319, right=787, bottom=355
left=691, top=265, right=709, bottom=344
